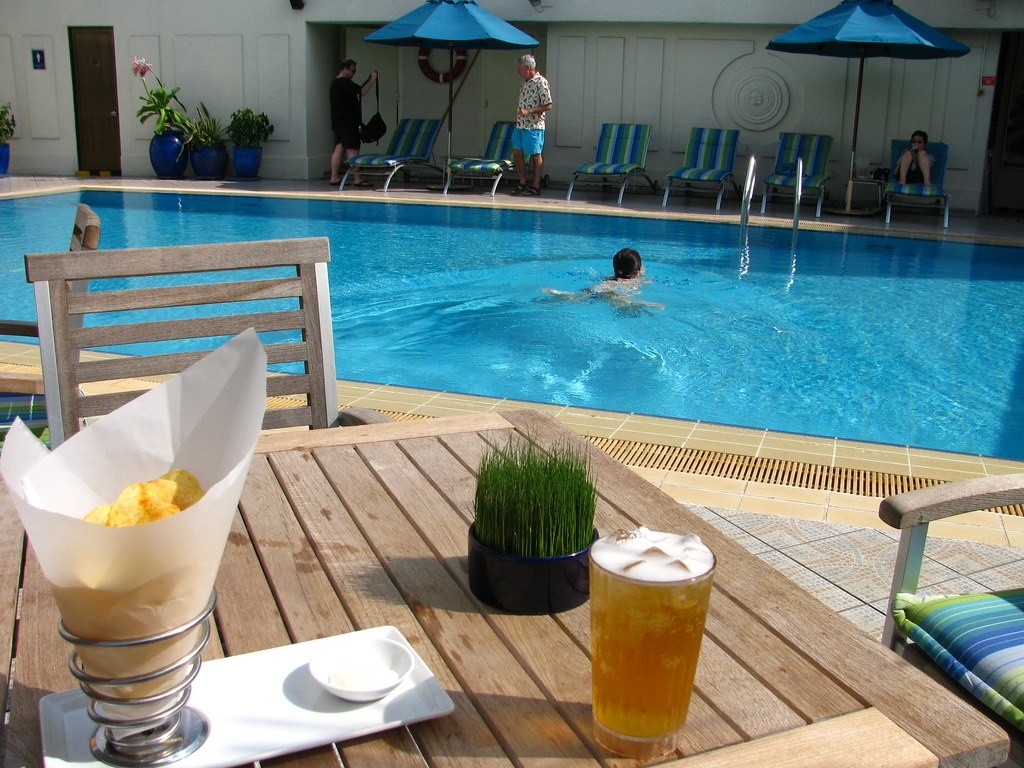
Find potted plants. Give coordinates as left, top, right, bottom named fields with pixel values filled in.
left=0, top=102, right=16, bottom=177
left=468, top=422, right=599, bottom=616
left=183, top=100, right=275, bottom=180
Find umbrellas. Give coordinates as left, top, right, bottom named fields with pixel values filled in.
left=765, top=0, right=972, bottom=180
left=363, top=0, right=539, bottom=158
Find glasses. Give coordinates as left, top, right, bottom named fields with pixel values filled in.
left=912, top=139, right=923, bottom=144
left=348, top=69, right=356, bottom=74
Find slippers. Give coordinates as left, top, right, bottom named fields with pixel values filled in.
left=354, top=180, right=375, bottom=187
left=330, top=180, right=351, bottom=186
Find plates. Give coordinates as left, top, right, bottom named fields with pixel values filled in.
left=38, top=626, right=456, bottom=768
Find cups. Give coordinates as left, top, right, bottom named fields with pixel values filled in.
left=589, top=536, right=718, bottom=759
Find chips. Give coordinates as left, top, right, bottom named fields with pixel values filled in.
left=76, top=470, right=206, bottom=527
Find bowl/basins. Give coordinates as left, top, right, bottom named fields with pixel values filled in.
left=307, top=638, right=414, bottom=702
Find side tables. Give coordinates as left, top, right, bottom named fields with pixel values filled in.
left=843, top=180, right=884, bottom=209
left=442, top=156, right=471, bottom=179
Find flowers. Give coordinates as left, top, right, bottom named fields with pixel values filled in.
left=130, top=56, right=188, bottom=135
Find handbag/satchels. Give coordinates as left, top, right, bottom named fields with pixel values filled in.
left=359, top=112, right=387, bottom=143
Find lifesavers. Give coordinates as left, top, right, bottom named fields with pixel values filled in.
left=418, top=44, right=468, bottom=83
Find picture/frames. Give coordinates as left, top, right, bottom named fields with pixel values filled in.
left=32, top=50, right=45, bottom=69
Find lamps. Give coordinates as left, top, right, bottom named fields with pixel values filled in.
left=975, top=0, right=996, bottom=19
left=529, top=0, right=554, bottom=13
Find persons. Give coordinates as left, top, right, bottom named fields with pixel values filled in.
left=510, top=54, right=553, bottom=196
left=895, top=130, right=933, bottom=185
left=329, top=58, right=377, bottom=186
left=614, top=248, right=645, bottom=279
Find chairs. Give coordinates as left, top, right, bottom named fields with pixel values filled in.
left=0, top=204, right=101, bottom=450
left=443, top=121, right=544, bottom=197
left=879, top=472, right=1024, bottom=768
left=761, top=132, right=833, bottom=218
left=884, top=140, right=949, bottom=228
left=567, top=123, right=659, bottom=204
left=662, top=127, right=741, bottom=210
left=339, top=119, right=453, bottom=193
left=24, top=237, right=400, bottom=452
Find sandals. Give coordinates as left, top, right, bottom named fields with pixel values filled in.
left=511, top=184, right=528, bottom=194
left=525, top=188, right=541, bottom=196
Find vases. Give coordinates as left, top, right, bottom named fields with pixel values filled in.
left=149, top=130, right=190, bottom=179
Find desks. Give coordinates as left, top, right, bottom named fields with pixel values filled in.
left=0, top=410, right=1024, bottom=768
